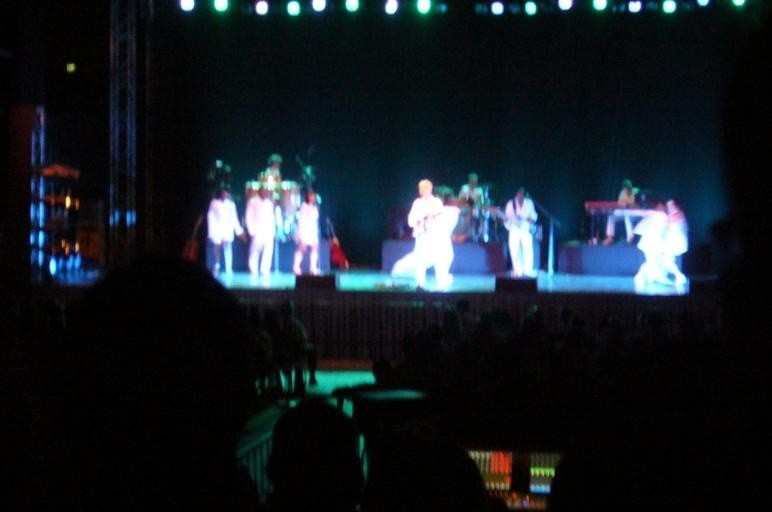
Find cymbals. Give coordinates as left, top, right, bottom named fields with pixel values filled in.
left=434, top=186, right=455, bottom=195
left=475, top=182, right=500, bottom=188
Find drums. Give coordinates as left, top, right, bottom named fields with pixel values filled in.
left=280, top=181, right=298, bottom=215
left=246, top=182, right=261, bottom=200
left=266, top=182, right=280, bottom=209
left=445, top=198, right=475, bottom=241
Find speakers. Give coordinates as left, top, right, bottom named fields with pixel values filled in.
left=294, top=274, right=338, bottom=289
left=495, top=274, right=538, bottom=294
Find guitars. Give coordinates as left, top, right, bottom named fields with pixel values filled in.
left=180, top=215, right=203, bottom=262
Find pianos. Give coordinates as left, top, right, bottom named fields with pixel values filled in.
left=587, top=202, right=664, bottom=214
